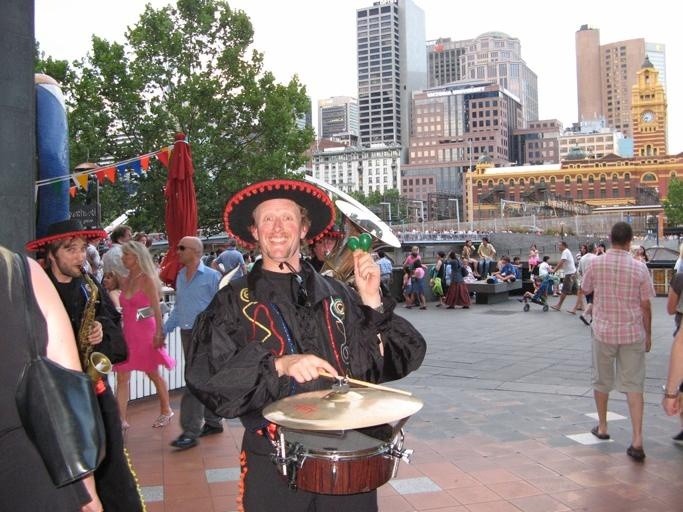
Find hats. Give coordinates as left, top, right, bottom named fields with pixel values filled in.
left=27, top=217, right=108, bottom=248
left=224, top=180, right=336, bottom=247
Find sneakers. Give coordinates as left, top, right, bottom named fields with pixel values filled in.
left=627, top=445, right=646, bottom=460
left=406, top=300, right=469, bottom=310
left=200, top=424, right=224, bottom=437
left=592, top=426, right=609, bottom=440
left=169, top=434, right=197, bottom=449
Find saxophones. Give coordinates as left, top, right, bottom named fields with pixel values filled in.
left=77, top=266, right=112, bottom=383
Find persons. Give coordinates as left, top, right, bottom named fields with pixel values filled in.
left=1, top=242, right=105, bottom=511
left=39, top=235, right=147, bottom=512
left=183, top=198, right=426, bottom=512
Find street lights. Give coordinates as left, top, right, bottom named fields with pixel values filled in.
left=434, top=137, right=478, bottom=232
left=71, top=161, right=104, bottom=232
left=377, top=198, right=465, bottom=235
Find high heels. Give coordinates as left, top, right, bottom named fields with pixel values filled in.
left=151, top=412, right=176, bottom=430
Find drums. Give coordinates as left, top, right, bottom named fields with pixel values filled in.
left=274, top=424, right=404, bottom=496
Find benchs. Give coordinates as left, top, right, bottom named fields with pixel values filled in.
left=466, top=278, right=524, bottom=305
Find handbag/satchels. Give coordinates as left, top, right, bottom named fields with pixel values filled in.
left=6, top=252, right=109, bottom=488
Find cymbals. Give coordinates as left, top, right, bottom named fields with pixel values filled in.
left=263, top=389, right=423, bottom=430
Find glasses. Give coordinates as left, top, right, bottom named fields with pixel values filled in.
left=176, top=245, right=195, bottom=252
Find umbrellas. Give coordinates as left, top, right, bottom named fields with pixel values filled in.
left=157, top=131, right=198, bottom=290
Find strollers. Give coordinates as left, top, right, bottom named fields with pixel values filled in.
left=519, top=269, right=551, bottom=313
